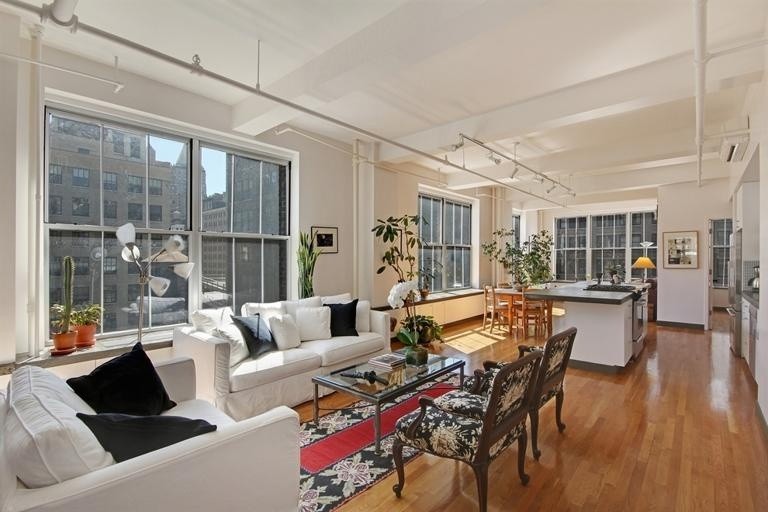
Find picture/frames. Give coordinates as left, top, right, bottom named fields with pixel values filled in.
left=662, top=230, right=698, bottom=270
left=312, top=224, right=339, bottom=256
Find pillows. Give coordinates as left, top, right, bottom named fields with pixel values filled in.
left=321, top=297, right=362, bottom=339
left=188, top=306, right=236, bottom=335
left=120, top=290, right=230, bottom=329
left=294, top=306, right=332, bottom=342
left=2, top=365, right=115, bottom=488
left=73, top=407, right=217, bottom=466
left=209, top=322, right=252, bottom=368
left=269, top=314, right=302, bottom=352
left=287, top=295, right=323, bottom=324
left=240, top=298, right=289, bottom=317
left=355, top=300, right=372, bottom=333
left=230, top=310, right=278, bottom=360
left=64, top=340, right=180, bottom=416
left=321, top=291, right=356, bottom=306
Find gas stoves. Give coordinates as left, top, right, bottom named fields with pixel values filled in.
left=583, top=284, right=636, bottom=292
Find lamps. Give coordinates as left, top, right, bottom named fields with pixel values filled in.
left=639, top=241, right=654, bottom=279
left=630, top=256, right=658, bottom=286
left=450, top=132, right=576, bottom=199
left=114, top=219, right=195, bottom=345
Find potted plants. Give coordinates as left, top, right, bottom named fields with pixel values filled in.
left=605, top=259, right=617, bottom=281
left=50, top=254, right=79, bottom=354
left=370, top=211, right=446, bottom=345
left=49, top=303, right=105, bottom=348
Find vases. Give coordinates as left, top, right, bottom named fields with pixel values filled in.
left=401, top=346, right=429, bottom=369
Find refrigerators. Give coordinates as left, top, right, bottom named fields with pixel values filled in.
left=725, top=227, right=742, bottom=361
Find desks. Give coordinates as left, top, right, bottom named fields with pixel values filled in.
left=487, top=283, right=550, bottom=336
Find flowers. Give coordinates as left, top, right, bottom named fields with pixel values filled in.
left=383, top=278, right=427, bottom=345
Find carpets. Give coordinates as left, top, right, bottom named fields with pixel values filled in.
left=295, top=368, right=476, bottom=511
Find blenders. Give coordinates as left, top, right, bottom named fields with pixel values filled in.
left=748, top=265, right=760, bottom=292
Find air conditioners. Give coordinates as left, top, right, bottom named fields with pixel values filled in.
left=717, top=113, right=750, bottom=163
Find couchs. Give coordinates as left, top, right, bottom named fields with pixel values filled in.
left=0, top=353, right=303, bottom=512
left=172, top=290, right=393, bottom=423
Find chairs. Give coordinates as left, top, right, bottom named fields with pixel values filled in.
left=463, top=325, right=579, bottom=462
left=389, top=348, right=546, bottom=512
left=488, top=291, right=515, bottom=336
left=481, top=285, right=502, bottom=332
left=515, top=287, right=547, bottom=340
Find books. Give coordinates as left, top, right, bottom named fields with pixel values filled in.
left=367, top=353, right=407, bottom=369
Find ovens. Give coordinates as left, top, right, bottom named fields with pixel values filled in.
left=632, top=289, right=646, bottom=342
